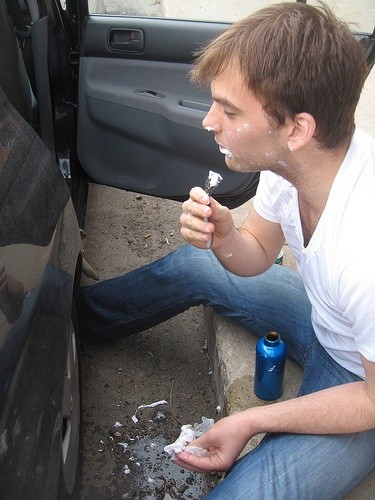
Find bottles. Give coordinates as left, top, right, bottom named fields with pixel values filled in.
left=254, top=331, right=287, bottom=400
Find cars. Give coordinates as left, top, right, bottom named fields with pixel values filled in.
left=1, top=0, right=374, bottom=500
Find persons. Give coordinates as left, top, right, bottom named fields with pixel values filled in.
left=78, top=0, right=375, bottom=500
